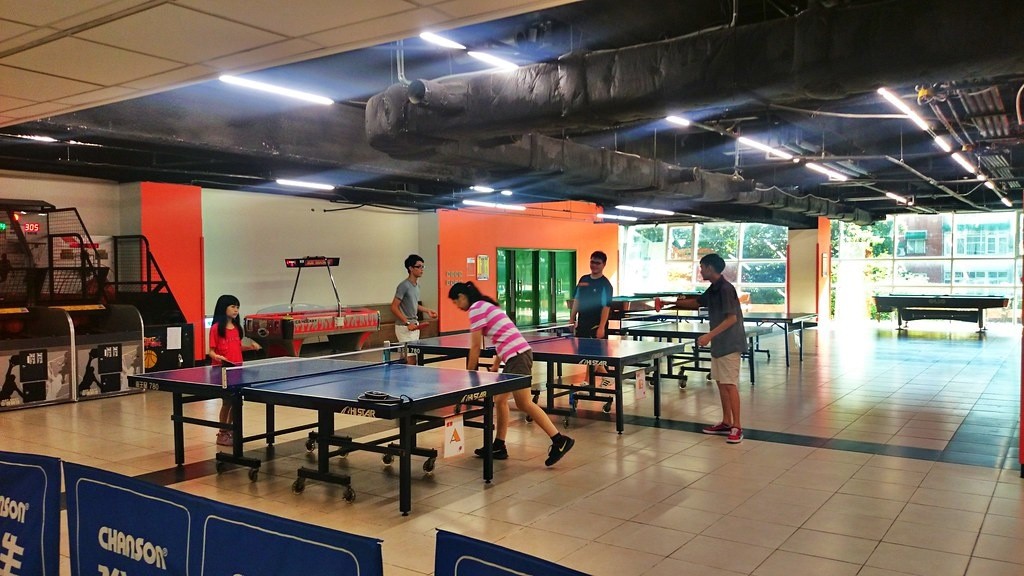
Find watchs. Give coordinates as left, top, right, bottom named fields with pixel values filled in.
left=405, top=321, right=411, bottom=326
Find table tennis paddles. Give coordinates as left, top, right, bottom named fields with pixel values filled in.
left=408, top=322, right=429, bottom=331
left=655, top=297, right=676, bottom=312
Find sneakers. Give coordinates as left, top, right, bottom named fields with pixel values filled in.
left=701, top=420, right=744, bottom=443
left=578, top=381, right=592, bottom=396
left=545, top=434, right=575, bottom=467
left=599, top=379, right=614, bottom=388
left=216, top=430, right=234, bottom=446
left=474, top=444, right=508, bottom=459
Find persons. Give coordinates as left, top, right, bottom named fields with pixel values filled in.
left=570, top=251, right=613, bottom=387
left=209, top=295, right=260, bottom=446
left=449, top=281, right=575, bottom=466
left=391, top=255, right=438, bottom=366
left=676, top=253, right=749, bottom=443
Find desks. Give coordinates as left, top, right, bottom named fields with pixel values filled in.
left=537, top=318, right=774, bottom=389
left=126, top=356, right=532, bottom=516
left=567, top=290, right=706, bottom=322
left=391, top=332, right=685, bottom=435
left=623, top=309, right=818, bottom=366
left=243, top=309, right=381, bottom=357
left=873, top=292, right=1010, bottom=334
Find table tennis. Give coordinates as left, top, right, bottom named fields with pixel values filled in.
left=433, top=314, right=436, bottom=318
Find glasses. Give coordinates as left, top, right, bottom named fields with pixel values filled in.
left=590, top=261, right=604, bottom=265
left=412, top=265, right=426, bottom=269
